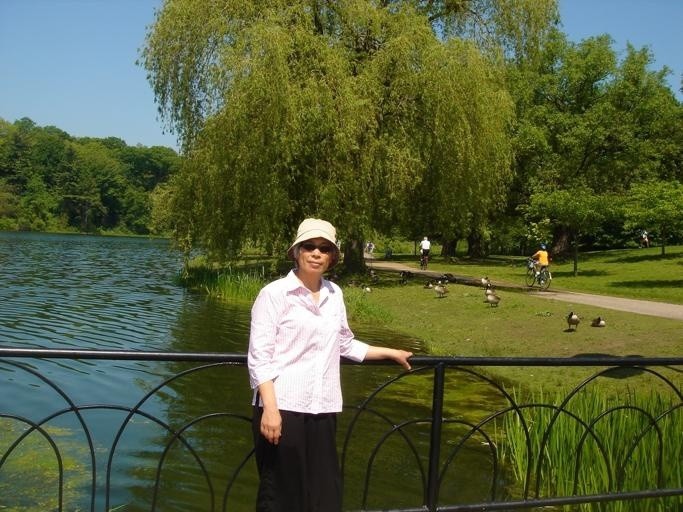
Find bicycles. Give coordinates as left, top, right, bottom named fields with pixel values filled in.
left=419, top=249, right=430, bottom=271
left=524, top=257, right=554, bottom=293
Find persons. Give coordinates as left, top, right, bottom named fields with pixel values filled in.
left=529, top=243, right=548, bottom=283
left=641, top=234, right=650, bottom=247
left=419, top=236, right=431, bottom=265
left=365, top=241, right=374, bottom=253
left=247, top=218, right=414, bottom=512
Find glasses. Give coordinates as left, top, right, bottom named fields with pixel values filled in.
left=298, top=243, right=335, bottom=254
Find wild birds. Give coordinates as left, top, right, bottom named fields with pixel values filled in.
left=591, top=317, right=606, bottom=327
left=481, top=276, right=501, bottom=308
left=423, top=272, right=450, bottom=299
left=566, top=312, right=580, bottom=329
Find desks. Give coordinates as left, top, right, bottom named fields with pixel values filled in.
left=488, top=255, right=529, bottom=267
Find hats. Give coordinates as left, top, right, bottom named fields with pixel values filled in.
left=285, top=217, right=341, bottom=272
